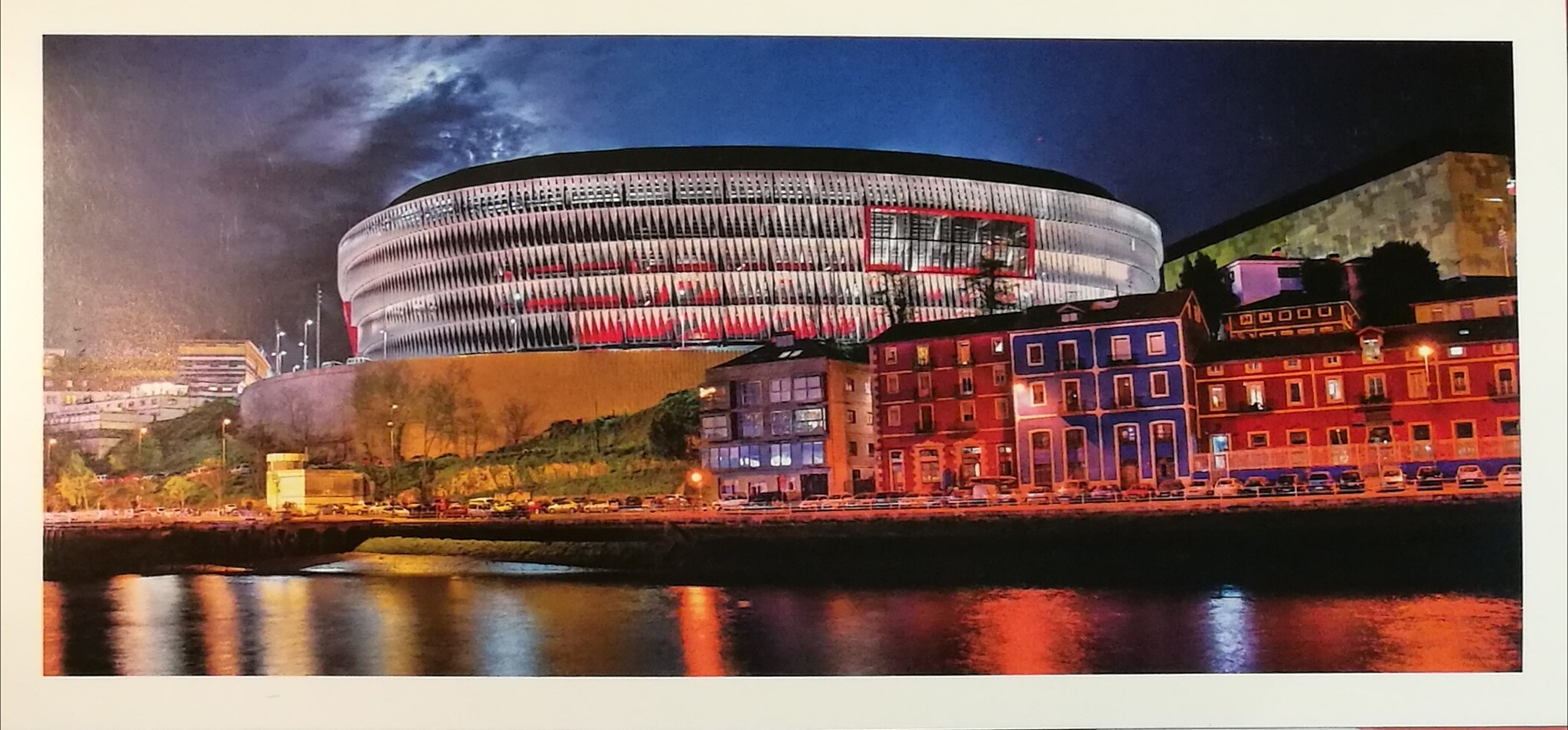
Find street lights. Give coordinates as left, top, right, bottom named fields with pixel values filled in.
left=222, top=419, right=230, bottom=467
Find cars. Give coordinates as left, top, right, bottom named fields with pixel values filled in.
left=1306, top=471, right=1335, bottom=495
left=1497, top=465, right=1522, bottom=486
left=1380, top=468, right=1406, bottom=492
left=1214, top=478, right=1243, bottom=497
left=1123, top=483, right=1155, bottom=502
left=1155, top=480, right=1185, bottom=501
left=229, top=463, right=252, bottom=474
left=897, top=480, right=1090, bottom=509
left=799, top=492, right=903, bottom=510
left=1242, top=476, right=1272, bottom=497
left=1090, top=484, right=1122, bottom=502
left=546, top=491, right=788, bottom=513
left=1336, top=470, right=1365, bottom=493
left=1414, top=466, right=1443, bottom=491
left=168, top=504, right=253, bottom=517
left=1185, top=479, right=1213, bottom=500
left=1455, top=465, right=1487, bottom=488
left=1273, top=474, right=1299, bottom=495
left=316, top=498, right=552, bottom=518
